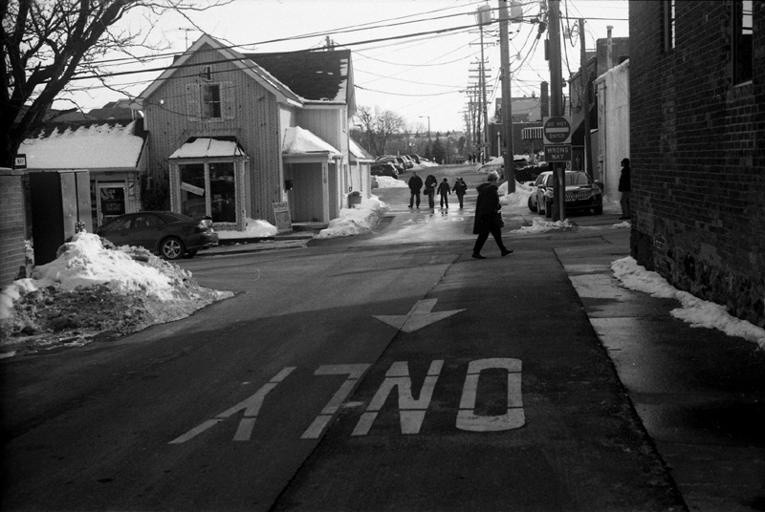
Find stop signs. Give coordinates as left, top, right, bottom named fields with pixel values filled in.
left=543, top=116, right=573, bottom=144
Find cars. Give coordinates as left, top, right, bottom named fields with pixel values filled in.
left=371, top=153, right=420, bottom=179
left=529, top=170, right=604, bottom=222
left=93, top=209, right=219, bottom=259
left=503, top=159, right=553, bottom=182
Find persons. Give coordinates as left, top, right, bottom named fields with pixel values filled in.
left=472, top=170, right=513, bottom=259
left=407, top=171, right=423, bottom=208
left=437, top=178, right=451, bottom=209
left=452, top=177, right=467, bottom=208
left=424, top=175, right=437, bottom=208
left=618, top=158, right=630, bottom=220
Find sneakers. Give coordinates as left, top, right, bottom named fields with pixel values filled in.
left=406, top=205, right=464, bottom=209
left=501, top=248, right=514, bottom=257
left=472, top=251, right=487, bottom=259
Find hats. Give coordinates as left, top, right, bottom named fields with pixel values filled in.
left=488, top=171, right=500, bottom=180
